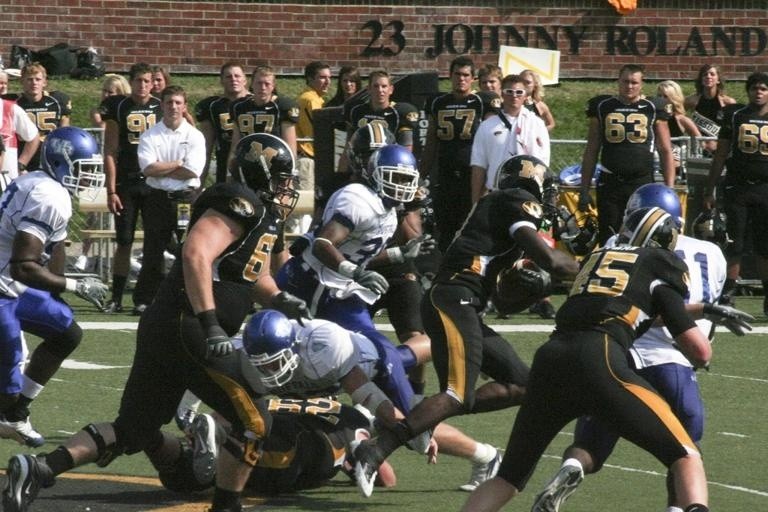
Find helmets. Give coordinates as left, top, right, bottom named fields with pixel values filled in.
left=494, top=155, right=557, bottom=221
left=42, top=127, right=106, bottom=200
left=346, top=125, right=420, bottom=207
left=617, top=183, right=682, bottom=251
left=693, top=207, right=735, bottom=250
left=230, top=134, right=300, bottom=219
left=563, top=213, right=600, bottom=255
left=242, top=310, right=302, bottom=387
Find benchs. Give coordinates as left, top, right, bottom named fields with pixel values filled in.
left=80, top=230, right=145, bottom=289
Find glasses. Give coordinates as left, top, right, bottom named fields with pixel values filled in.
left=505, top=89, right=526, bottom=96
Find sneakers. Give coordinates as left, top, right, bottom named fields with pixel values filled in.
left=193, top=414, right=217, bottom=484
left=531, top=465, right=584, bottom=512
left=539, top=301, right=559, bottom=319
left=345, top=439, right=381, bottom=499
left=459, top=448, right=503, bottom=491
left=0, top=452, right=52, bottom=511
left=0, top=414, right=45, bottom=448
left=410, top=394, right=437, bottom=455
left=175, top=389, right=202, bottom=433
left=133, top=304, right=147, bottom=314
left=102, top=301, right=123, bottom=313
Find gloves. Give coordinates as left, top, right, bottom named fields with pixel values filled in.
left=579, top=186, right=596, bottom=213
left=338, top=260, right=390, bottom=294
left=387, top=233, right=435, bottom=265
left=64, top=277, right=109, bottom=311
left=702, top=304, right=755, bottom=336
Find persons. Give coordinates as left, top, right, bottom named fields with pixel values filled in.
left=1, top=54, right=767, bottom=511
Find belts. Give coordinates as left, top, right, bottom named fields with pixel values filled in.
left=146, top=187, right=192, bottom=198
